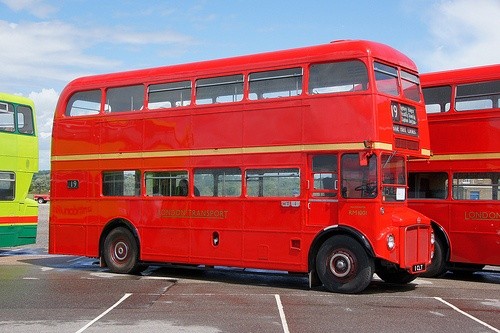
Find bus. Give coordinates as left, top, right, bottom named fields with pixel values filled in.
left=48, top=38, right=436, bottom=295
left=348, top=64, right=498, bottom=278
left=0, top=90, right=38, bottom=248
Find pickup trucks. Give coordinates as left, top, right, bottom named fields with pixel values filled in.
left=32, top=188, right=51, bottom=204
